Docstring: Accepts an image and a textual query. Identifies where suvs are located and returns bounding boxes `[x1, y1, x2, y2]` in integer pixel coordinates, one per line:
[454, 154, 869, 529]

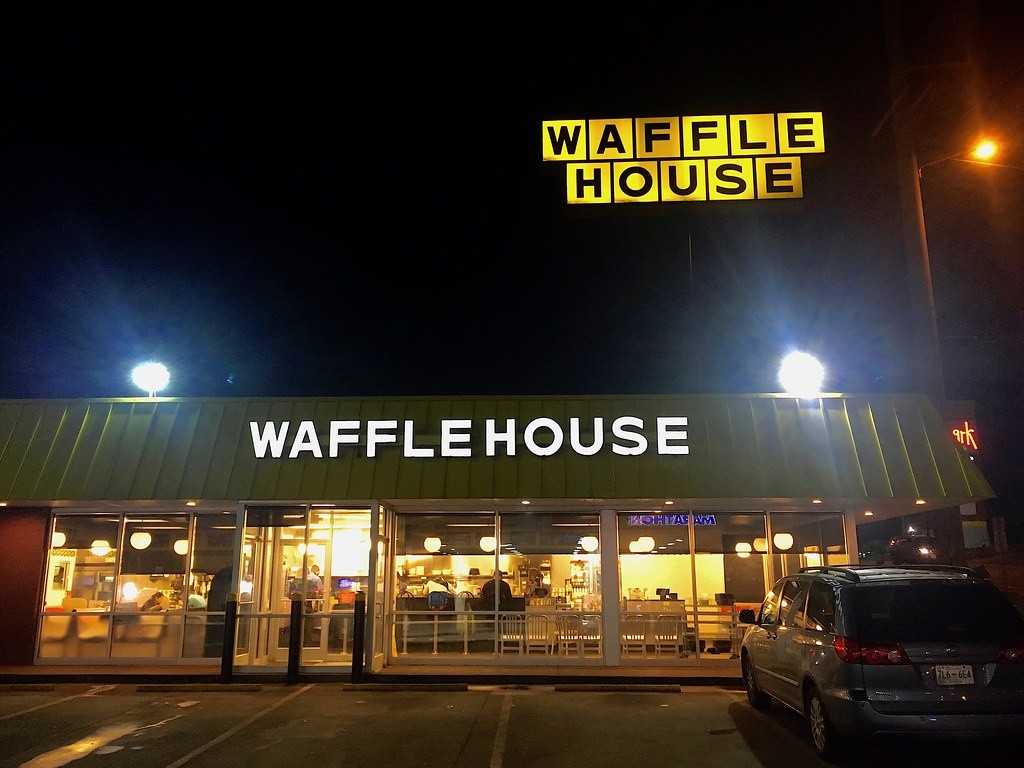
[740, 564, 1024, 760]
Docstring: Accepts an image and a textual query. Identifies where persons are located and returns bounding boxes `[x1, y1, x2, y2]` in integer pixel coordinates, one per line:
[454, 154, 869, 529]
[288, 567, 318, 601]
[178, 592, 205, 609]
[306, 565, 324, 596]
[422, 575, 457, 614]
[139, 592, 164, 613]
[480, 570, 513, 633]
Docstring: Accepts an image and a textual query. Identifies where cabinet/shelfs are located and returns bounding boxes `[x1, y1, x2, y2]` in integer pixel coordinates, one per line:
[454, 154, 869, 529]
[681, 603, 740, 659]
[593, 569, 621, 601]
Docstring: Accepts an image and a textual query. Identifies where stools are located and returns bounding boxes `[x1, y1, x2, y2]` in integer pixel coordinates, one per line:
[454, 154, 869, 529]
[456, 591, 474, 655]
[426, 591, 449, 655]
[397, 591, 415, 655]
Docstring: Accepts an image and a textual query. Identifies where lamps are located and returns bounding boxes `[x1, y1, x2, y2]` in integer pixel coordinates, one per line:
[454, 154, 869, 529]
[52, 531, 66, 549]
[130, 519, 151, 550]
[480, 524, 497, 552]
[173, 539, 188, 556]
[582, 524, 598, 552]
[423, 538, 440, 552]
[637, 536, 655, 552]
[735, 532, 793, 558]
[628, 540, 637, 552]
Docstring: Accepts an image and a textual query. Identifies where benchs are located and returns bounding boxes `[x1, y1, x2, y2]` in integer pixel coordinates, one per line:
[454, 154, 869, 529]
[41, 608, 169, 658]
[115, 602, 137, 608]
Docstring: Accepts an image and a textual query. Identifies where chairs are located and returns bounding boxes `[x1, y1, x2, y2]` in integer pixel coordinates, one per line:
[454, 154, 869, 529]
[654, 615, 679, 657]
[526, 615, 548, 654]
[501, 614, 523, 655]
[622, 615, 647, 658]
[580, 615, 602, 657]
[557, 615, 580, 657]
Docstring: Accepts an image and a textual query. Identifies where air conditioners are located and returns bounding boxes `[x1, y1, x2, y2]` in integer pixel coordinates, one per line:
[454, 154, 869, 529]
[89, 540, 111, 556]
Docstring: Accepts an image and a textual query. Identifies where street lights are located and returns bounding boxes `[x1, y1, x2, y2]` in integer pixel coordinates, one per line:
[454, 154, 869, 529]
[899, 132, 1008, 400]
